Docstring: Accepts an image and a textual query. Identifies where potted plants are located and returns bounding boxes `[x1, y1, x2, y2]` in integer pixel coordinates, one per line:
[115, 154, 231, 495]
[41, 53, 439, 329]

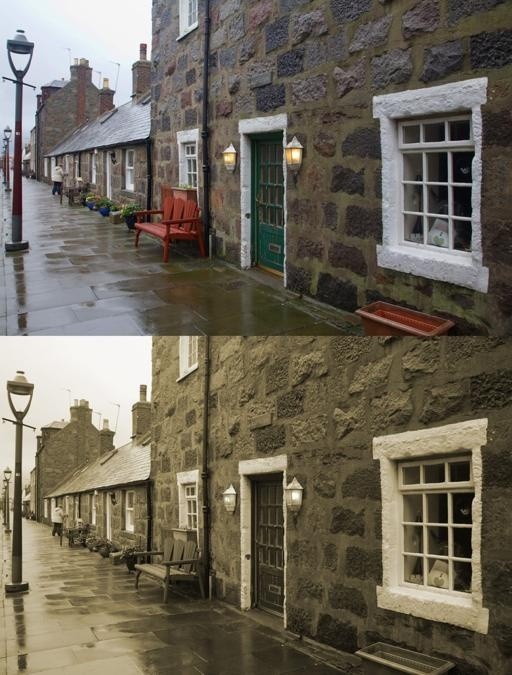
[79, 191, 141, 230]
[86, 536, 145, 575]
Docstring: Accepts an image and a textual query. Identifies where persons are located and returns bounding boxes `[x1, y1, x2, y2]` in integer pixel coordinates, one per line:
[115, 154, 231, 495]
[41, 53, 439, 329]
[51, 504, 63, 536]
[52, 162, 66, 196]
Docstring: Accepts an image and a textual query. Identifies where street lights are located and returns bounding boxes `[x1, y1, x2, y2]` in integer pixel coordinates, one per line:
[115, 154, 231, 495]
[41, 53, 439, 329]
[5, 592, 33, 675]
[2, 465, 12, 533]
[2, 124, 13, 193]
[1, 370, 39, 594]
[5, 250, 33, 336]
[0, 27, 37, 253]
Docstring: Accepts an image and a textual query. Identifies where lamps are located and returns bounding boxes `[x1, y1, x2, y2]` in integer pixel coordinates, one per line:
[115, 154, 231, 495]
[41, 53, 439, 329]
[284, 476, 304, 520]
[223, 483, 236, 515]
[285, 134, 303, 182]
[223, 141, 237, 173]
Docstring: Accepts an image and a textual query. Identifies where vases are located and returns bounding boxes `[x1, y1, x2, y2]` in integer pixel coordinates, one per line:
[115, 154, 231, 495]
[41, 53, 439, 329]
[357, 300, 456, 336]
[353, 641, 456, 675]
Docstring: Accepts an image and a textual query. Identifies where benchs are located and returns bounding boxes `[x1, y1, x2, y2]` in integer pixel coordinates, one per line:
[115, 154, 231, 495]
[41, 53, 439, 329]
[132, 197, 206, 263]
[134, 539, 206, 604]
